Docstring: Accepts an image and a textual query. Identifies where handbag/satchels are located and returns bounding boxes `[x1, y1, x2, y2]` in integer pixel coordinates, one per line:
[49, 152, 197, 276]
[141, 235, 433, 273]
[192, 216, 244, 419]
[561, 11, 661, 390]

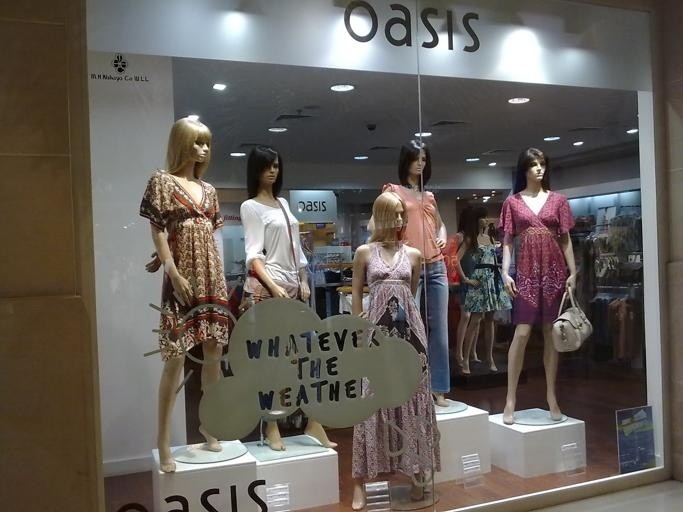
[551, 286, 593, 353]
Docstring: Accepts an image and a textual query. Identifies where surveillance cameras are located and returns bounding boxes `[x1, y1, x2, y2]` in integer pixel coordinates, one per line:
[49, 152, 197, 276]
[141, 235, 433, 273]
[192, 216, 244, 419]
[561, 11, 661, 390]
[367, 121, 377, 130]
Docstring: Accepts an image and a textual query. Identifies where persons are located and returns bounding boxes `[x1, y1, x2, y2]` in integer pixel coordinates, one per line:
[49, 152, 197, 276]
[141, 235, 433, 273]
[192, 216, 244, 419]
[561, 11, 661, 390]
[382, 141, 452, 408]
[453, 224, 482, 369]
[346, 190, 440, 511]
[455, 201, 501, 374]
[140, 115, 229, 474]
[496, 149, 578, 422]
[240, 142, 339, 449]
